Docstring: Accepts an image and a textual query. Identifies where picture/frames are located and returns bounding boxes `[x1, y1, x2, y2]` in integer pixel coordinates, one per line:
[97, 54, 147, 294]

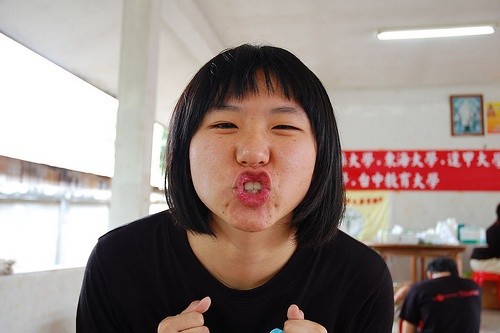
[450, 94, 484, 136]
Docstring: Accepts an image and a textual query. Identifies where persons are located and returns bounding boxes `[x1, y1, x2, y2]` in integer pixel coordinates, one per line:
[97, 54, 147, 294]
[397, 256, 481, 333]
[75, 43, 393, 333]
[486, 204, 500, 248]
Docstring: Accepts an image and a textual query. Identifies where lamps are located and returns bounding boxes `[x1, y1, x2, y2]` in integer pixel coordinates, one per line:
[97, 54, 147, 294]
[375, 21, 496, 41]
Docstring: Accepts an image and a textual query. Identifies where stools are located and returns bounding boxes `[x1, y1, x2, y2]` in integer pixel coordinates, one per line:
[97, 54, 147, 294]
[473, 271, 500, 311]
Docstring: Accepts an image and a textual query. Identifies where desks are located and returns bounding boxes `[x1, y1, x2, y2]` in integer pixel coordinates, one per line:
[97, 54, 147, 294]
[366, 243, 466, 286]
